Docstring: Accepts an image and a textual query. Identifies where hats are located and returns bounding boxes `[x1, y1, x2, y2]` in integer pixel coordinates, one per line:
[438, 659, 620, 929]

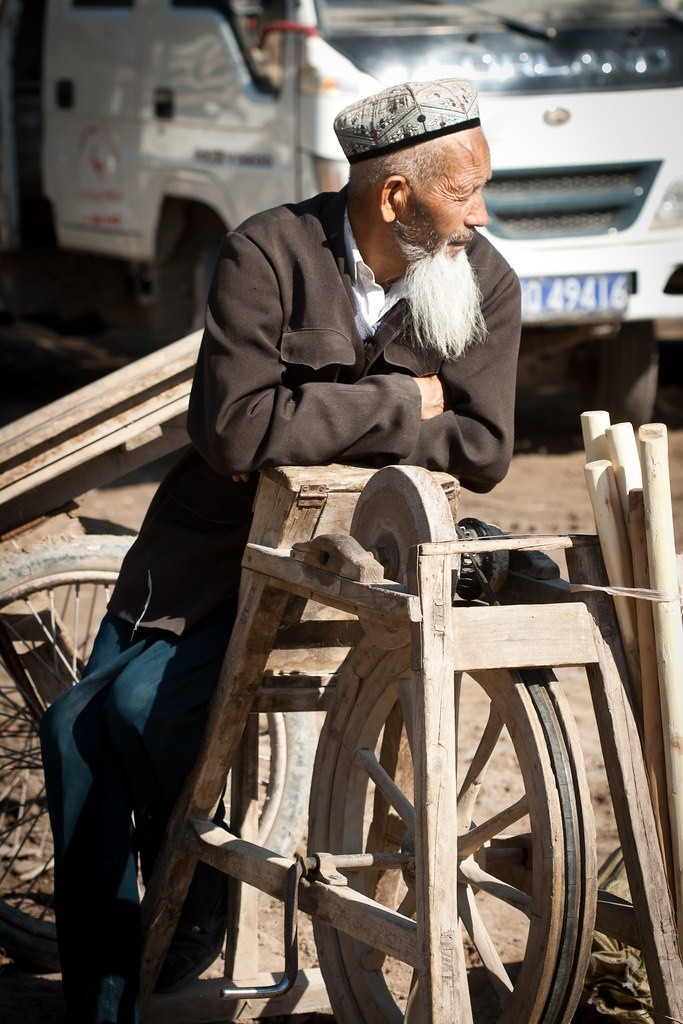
[333, 79, 480, 164]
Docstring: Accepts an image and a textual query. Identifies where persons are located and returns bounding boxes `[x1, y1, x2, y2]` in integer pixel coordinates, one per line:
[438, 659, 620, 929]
[38, 77, 522, 1024]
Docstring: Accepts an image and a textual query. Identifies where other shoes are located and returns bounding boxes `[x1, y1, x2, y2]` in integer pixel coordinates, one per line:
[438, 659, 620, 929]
[152, 830, 241, 994]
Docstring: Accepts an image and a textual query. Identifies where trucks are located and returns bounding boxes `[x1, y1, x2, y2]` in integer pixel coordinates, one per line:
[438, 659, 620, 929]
[2, 0, 683, 433]
